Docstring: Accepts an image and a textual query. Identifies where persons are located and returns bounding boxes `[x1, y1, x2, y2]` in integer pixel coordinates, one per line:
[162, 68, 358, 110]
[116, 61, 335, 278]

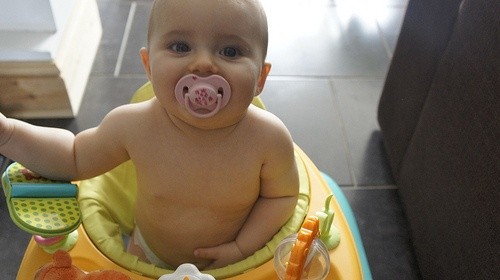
[0, 0, 299, 271]
